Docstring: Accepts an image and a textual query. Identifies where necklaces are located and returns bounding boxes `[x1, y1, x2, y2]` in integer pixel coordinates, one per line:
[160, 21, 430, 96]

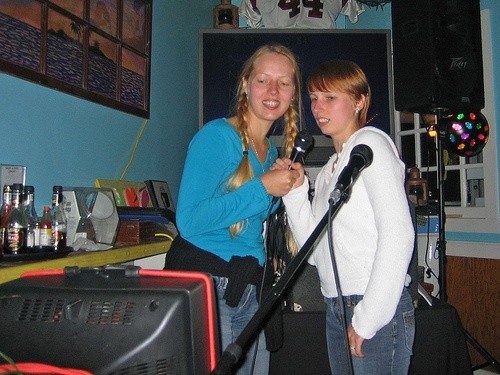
[249, 134, 269, 175]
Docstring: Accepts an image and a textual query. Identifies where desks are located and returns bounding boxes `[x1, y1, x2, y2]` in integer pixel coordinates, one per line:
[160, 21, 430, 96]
[269, 296, 474, 375]
[0, 241, 172, 284]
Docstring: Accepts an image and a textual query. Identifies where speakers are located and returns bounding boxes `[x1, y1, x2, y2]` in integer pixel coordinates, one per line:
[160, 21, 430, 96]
[391, 0, 485, 117]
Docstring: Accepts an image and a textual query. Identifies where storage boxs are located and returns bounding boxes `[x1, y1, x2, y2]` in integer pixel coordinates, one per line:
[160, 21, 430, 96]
[87, 220, 154, 244]
[144, 180, 176, 213]
[95, 178, 153, 207]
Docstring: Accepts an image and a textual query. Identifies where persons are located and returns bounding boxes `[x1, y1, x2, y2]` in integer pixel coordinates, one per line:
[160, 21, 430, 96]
[162, 44, 303, 374]
[270, 59, 415, 375]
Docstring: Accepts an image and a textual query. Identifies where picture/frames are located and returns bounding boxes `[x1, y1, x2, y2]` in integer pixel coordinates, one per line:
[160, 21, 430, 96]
[0, 0, 153, 120]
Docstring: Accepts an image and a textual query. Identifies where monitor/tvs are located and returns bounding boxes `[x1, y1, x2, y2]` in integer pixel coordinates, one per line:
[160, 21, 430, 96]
[0, 265, 221, 375]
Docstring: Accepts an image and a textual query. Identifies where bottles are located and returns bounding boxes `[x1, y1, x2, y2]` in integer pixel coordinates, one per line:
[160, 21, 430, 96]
[0, 185, 13, 254]
[40, 207, 52, 250]
[4, 184, 28, 255]
[51, 186, 67, 252]
[24, 186, 40, 252]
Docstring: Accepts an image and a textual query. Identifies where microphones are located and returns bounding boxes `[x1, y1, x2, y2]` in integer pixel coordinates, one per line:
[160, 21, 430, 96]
[328, 145, 373, 205]
[272, 131, 313, 204]
